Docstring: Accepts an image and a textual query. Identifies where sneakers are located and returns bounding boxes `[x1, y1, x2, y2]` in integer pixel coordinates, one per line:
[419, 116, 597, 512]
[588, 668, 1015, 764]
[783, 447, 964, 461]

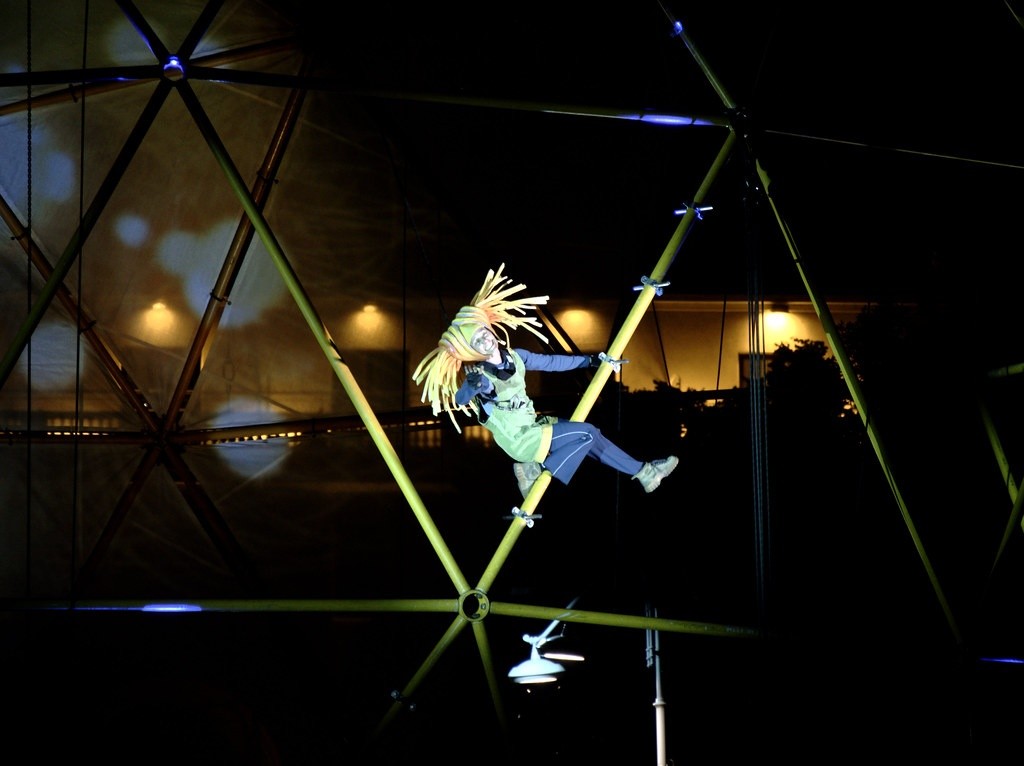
[514, 461, 542, 500]
[632, 456, 679, 493]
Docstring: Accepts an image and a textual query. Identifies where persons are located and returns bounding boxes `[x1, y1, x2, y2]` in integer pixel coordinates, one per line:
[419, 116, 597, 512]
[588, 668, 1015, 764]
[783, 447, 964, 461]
[415, 262, 680, 499]
